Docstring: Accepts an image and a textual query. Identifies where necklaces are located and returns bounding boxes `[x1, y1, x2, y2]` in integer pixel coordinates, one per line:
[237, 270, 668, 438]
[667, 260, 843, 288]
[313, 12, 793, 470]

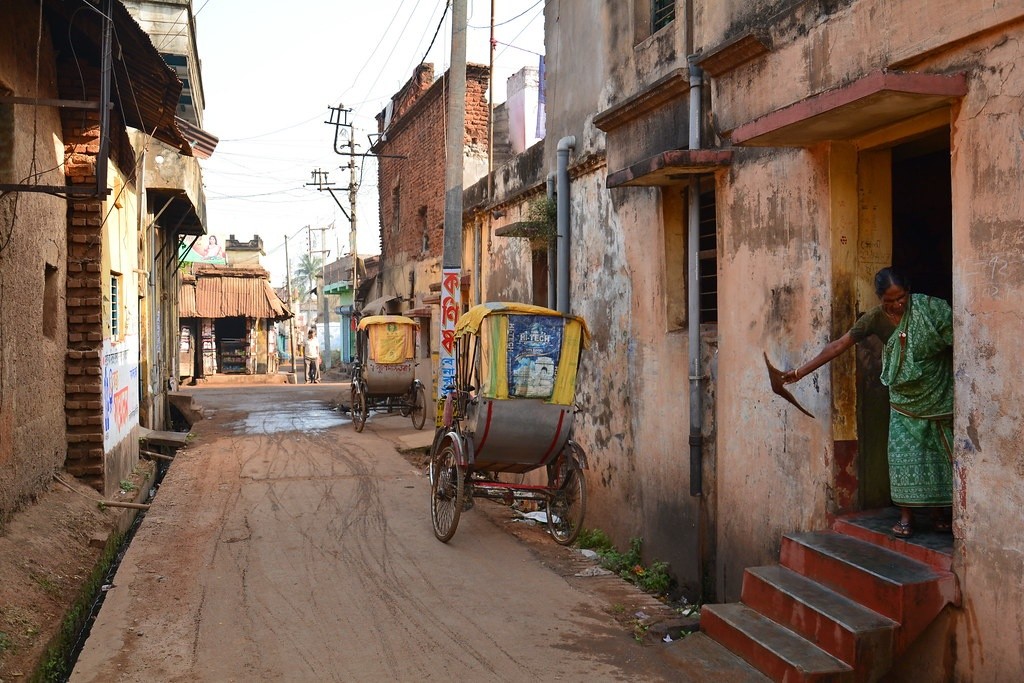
[890, 311, 911, 365]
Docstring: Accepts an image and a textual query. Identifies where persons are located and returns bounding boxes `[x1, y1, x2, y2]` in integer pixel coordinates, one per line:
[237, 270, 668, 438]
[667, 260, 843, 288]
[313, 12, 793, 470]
[302, 328, 321, 384]
[201, 235, 226, 265]
[780, 266, 953, 537]
[536, 366, 553, 385]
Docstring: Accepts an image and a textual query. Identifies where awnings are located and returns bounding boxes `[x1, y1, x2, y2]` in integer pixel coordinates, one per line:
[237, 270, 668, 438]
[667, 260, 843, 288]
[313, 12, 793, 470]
[147, 188, 206, 278]
[360, 295, 399, 316]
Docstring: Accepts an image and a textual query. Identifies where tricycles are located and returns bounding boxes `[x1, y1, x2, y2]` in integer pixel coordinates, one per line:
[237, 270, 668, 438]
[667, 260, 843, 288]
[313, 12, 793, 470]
[427, 302, 591, 545]
[349, 315, 429, 434]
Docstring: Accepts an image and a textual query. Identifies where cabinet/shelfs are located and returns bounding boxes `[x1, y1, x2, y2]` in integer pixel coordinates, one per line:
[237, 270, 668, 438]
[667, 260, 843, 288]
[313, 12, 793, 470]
[221, 338, 247, 373]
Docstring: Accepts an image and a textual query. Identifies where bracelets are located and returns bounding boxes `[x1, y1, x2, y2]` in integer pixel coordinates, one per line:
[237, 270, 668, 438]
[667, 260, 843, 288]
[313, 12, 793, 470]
[794, 368, 799, 378]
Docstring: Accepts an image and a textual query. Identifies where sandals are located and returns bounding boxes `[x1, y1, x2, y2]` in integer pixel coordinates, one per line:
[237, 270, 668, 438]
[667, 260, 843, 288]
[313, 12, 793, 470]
[931, 517, 951, 531]
[893, 517, 915, 538]
